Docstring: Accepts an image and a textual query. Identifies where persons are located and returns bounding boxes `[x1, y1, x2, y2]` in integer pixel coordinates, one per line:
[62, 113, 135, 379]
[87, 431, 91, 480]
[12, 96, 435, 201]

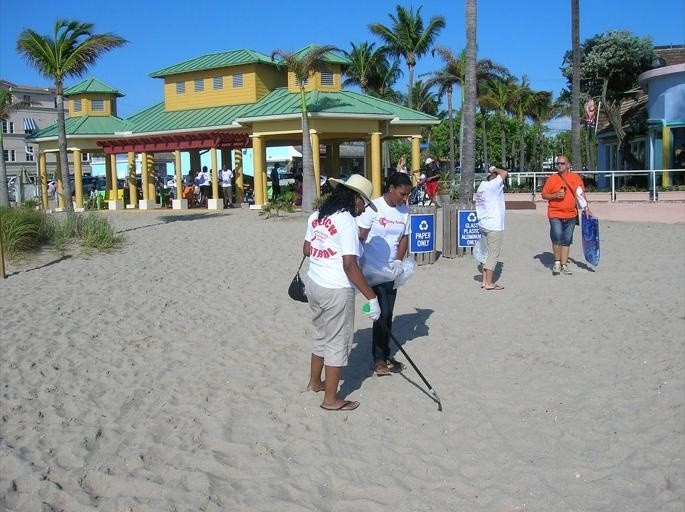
[397, 156, 409, 206]
[476, 166, 508, 291]
[88, 187, 100, 208]
[303, 174, 381, 411]
[542, 155, 593, 275]
[155, 163, 234, 209]
[270, 163, 280, 204]
[425, 157, 440, 208]
[355, 172, 413, 376]
[295, 178, 302, 206]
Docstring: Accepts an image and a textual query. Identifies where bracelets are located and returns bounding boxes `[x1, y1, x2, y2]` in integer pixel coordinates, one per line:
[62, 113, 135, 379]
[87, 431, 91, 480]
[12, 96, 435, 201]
[552, 194, 554, 199]
[584, 207, 589, 210]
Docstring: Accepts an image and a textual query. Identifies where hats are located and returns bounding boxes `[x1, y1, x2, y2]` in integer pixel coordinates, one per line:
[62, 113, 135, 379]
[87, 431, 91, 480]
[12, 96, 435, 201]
[328, 174, 377, 211]
[425, 157, 432, 165]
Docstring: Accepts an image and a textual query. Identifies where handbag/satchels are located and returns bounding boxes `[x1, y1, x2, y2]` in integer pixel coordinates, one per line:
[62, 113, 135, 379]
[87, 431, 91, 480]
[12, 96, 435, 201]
[582, 210, 599, 266]
[289, 270, 308, 302]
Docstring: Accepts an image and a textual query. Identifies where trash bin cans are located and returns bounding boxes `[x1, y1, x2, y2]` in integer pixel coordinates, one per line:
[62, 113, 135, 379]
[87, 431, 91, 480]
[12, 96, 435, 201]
[410, 204, 480, 265]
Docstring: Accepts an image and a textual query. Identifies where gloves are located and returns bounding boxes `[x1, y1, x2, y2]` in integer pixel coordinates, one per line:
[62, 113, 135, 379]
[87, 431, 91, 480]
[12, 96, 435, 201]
[365, 296, 382, 320]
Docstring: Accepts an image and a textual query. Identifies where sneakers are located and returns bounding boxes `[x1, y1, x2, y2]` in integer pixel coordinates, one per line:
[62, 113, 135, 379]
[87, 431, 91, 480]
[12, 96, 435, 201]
[553, 263, 572, 274]
[374, 358, 405, 375]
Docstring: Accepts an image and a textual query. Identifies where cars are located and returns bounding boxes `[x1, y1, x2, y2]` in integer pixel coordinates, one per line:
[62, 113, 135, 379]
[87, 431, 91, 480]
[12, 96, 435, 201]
[231, 175, 254, 198]
[70, 176, 175, 197]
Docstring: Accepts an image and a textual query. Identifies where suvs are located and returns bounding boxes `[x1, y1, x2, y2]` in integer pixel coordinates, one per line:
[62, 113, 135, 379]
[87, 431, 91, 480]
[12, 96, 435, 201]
[454, 166, 492, 181]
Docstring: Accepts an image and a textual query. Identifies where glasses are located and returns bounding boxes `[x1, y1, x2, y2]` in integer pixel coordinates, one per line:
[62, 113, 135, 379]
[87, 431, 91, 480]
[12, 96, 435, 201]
[556, 162, 565, 165]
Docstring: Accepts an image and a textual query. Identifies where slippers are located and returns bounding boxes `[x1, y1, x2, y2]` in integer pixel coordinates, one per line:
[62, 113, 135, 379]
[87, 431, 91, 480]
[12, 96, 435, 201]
[481, 284, 504, 290]
[320, 401, 359, 410]
